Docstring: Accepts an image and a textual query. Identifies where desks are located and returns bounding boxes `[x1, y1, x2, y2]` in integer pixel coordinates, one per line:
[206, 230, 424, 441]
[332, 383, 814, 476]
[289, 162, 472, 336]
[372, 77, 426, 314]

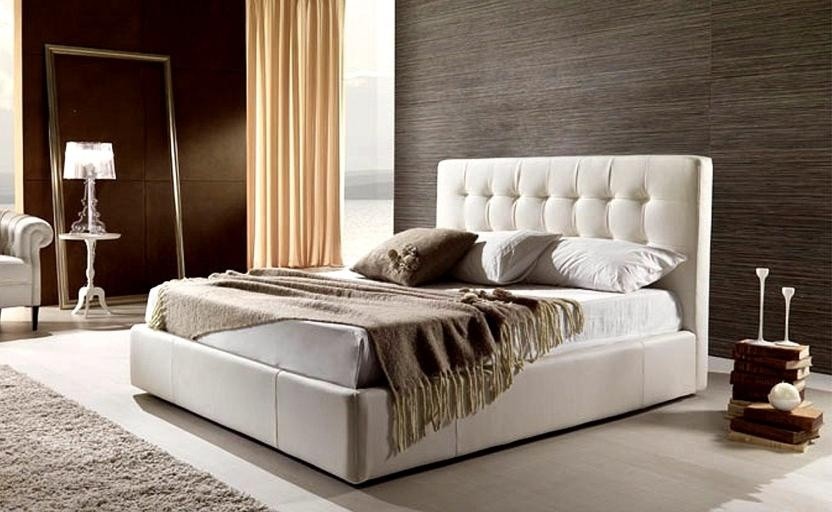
[58, 233, 122, 320]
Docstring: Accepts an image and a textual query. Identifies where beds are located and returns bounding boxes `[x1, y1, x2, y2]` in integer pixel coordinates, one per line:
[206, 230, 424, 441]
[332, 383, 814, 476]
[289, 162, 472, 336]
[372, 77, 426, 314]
[128, 154, 714, 487]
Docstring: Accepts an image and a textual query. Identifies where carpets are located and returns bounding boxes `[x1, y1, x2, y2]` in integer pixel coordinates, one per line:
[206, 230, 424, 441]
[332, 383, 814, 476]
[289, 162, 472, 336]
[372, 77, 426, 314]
[0, 363, 273, 512]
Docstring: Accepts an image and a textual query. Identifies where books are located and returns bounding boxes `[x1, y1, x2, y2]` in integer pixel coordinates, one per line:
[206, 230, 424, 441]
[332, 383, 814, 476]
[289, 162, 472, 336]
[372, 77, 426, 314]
[726, 337, 824, 453]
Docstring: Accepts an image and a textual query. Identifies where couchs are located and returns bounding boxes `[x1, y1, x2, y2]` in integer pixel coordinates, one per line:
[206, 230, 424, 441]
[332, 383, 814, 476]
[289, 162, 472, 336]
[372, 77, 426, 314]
[0, 208, 54, 334]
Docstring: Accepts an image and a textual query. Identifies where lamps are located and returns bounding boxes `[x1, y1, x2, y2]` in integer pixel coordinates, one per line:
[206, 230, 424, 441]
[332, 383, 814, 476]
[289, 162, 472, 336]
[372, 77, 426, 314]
[63, 142, 117, 233]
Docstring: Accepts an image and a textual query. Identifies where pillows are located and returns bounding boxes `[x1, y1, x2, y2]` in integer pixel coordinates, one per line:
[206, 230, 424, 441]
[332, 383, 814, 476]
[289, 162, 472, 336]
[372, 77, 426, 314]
[349, 228, 478, 287]
[525, 235, 689, 294]
[455, 230, 563, 285]
